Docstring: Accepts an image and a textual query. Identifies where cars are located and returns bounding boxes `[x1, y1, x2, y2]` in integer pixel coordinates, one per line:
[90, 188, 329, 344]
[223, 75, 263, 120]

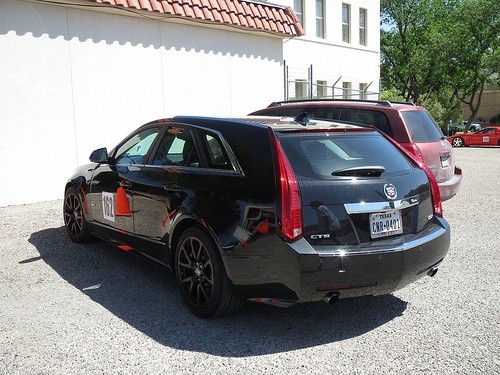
[445, 126, 499, 148]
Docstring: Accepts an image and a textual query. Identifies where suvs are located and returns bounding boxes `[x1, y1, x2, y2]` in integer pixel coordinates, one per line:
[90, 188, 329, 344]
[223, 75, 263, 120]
[62, 115, 453, 322]
[448, 120, 482, 133]
[246, 95, 462, 203]
